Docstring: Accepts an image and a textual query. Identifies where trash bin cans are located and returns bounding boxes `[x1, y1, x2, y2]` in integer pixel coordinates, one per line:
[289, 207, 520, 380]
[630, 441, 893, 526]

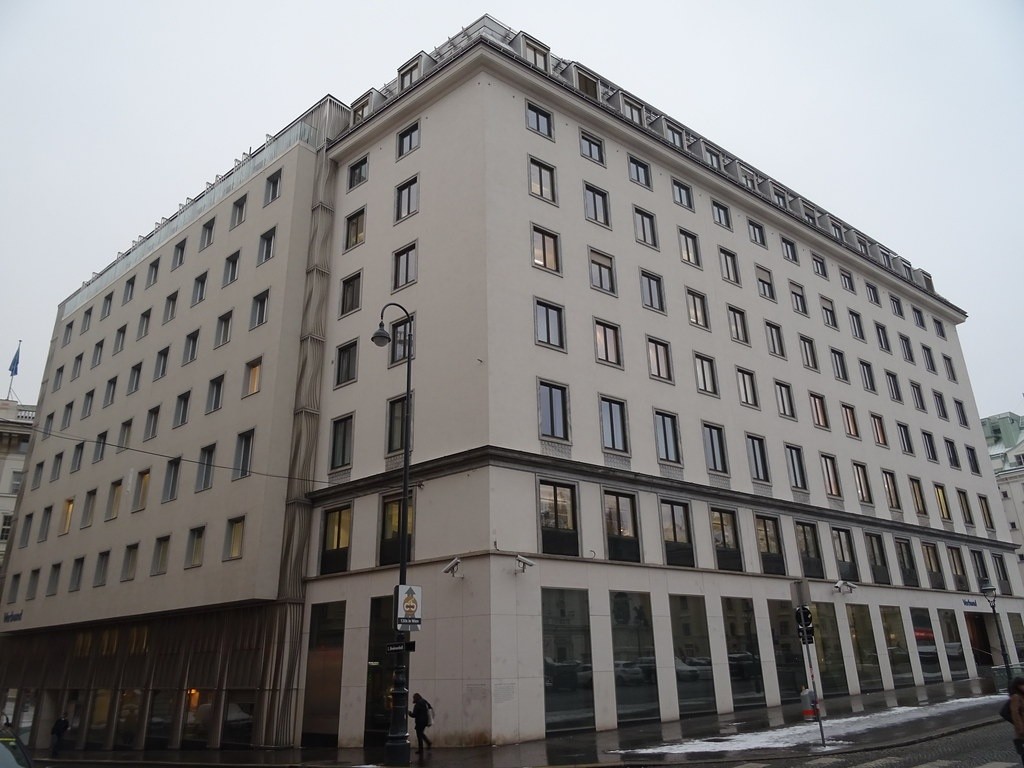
[800, 688, 820, 722]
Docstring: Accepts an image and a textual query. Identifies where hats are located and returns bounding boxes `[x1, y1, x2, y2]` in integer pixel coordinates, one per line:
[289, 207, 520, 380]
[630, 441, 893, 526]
[413, 693, 422, 702]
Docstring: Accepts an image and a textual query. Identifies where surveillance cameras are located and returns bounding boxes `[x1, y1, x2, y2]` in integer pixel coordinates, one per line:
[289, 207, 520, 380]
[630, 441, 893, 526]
[442, 558, 460, 573]
[515, 555, 535, 566]
[846, 582, 857, 588]
[835, 581, 843, 588]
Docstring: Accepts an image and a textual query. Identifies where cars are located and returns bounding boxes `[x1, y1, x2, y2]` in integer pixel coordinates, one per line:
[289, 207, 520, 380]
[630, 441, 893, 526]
[687, 652, 759, 681]
[579, 664, 594, 684]
[545, 655, 578, 690]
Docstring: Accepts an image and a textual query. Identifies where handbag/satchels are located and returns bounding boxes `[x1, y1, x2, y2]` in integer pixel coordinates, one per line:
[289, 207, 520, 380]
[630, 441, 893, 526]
[426, 701, 433, 728]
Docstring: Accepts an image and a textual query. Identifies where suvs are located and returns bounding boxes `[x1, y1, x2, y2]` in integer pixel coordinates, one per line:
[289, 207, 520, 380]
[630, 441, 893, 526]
[639, 656, 700, 681]
[614, 661, 643, 686]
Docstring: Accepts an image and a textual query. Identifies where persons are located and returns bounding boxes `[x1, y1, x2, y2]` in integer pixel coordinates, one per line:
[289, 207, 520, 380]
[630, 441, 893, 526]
[1008, 674, 1024, 768]
[50, 711, 70, 756]
[408, 693, 435, 754]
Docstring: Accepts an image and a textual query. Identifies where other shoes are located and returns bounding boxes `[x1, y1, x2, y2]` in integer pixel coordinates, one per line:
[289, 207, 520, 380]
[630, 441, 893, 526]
[415, 748, 424, 754]
[425, 741, 432, 750]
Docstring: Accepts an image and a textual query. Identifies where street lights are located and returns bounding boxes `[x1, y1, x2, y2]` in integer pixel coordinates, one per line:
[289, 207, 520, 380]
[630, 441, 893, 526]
[982, 577, 1013, 680]
[370, 304, 415, 768]
